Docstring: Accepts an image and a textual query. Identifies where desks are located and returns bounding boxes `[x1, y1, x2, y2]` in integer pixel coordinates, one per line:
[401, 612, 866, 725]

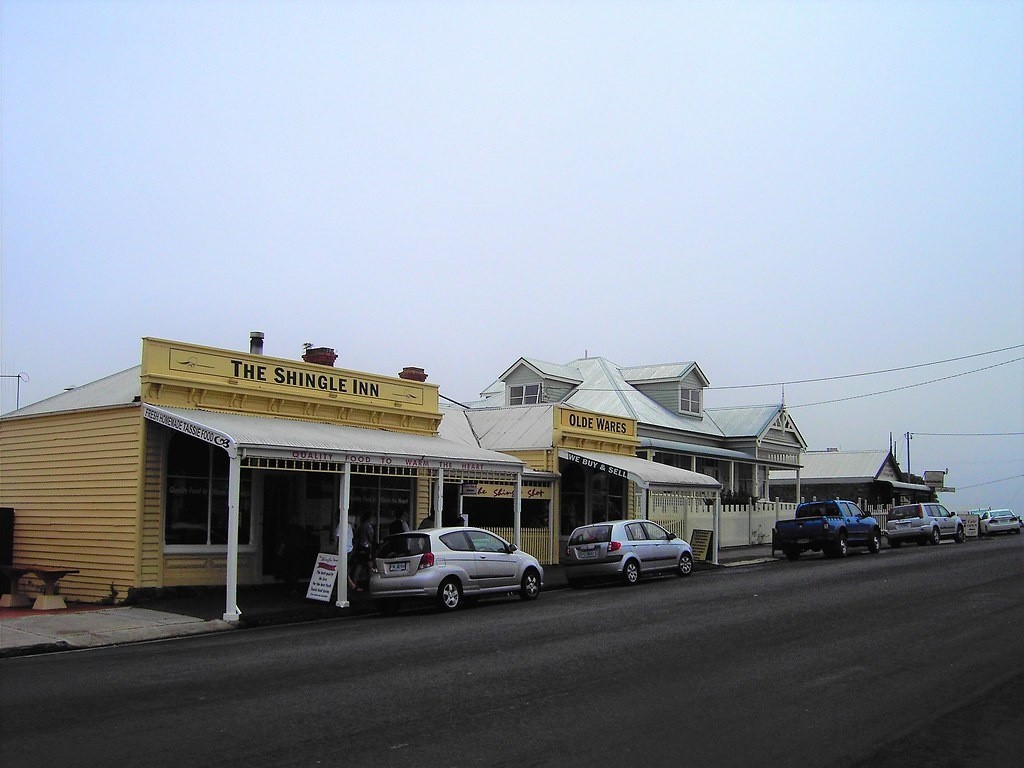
[0, 564, 80, 610]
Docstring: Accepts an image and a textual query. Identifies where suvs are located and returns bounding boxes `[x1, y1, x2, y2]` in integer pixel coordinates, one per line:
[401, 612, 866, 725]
[884, 503, 966, 546]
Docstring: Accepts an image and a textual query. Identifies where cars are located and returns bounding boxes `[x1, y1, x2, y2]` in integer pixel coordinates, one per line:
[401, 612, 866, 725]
[968, 509, 990, 521]
[564, 519, 695, 584]
[979, 509, 1020, 536]
[371, 527, 545, 610]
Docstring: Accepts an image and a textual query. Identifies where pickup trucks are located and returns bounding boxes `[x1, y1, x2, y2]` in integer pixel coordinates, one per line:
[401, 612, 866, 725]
[775, 500, 881, 558]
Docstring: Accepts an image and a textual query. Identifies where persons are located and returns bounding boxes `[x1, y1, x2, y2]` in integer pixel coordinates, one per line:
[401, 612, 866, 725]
[390, 510, 443, 554]
[335, 511, 376, 594]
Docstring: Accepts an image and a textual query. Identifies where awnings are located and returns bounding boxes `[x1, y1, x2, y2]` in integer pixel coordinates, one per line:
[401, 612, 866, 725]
[144, 401, 521, 621]
[558, 447, 725, 564]
[874, 479, 931, 494]
